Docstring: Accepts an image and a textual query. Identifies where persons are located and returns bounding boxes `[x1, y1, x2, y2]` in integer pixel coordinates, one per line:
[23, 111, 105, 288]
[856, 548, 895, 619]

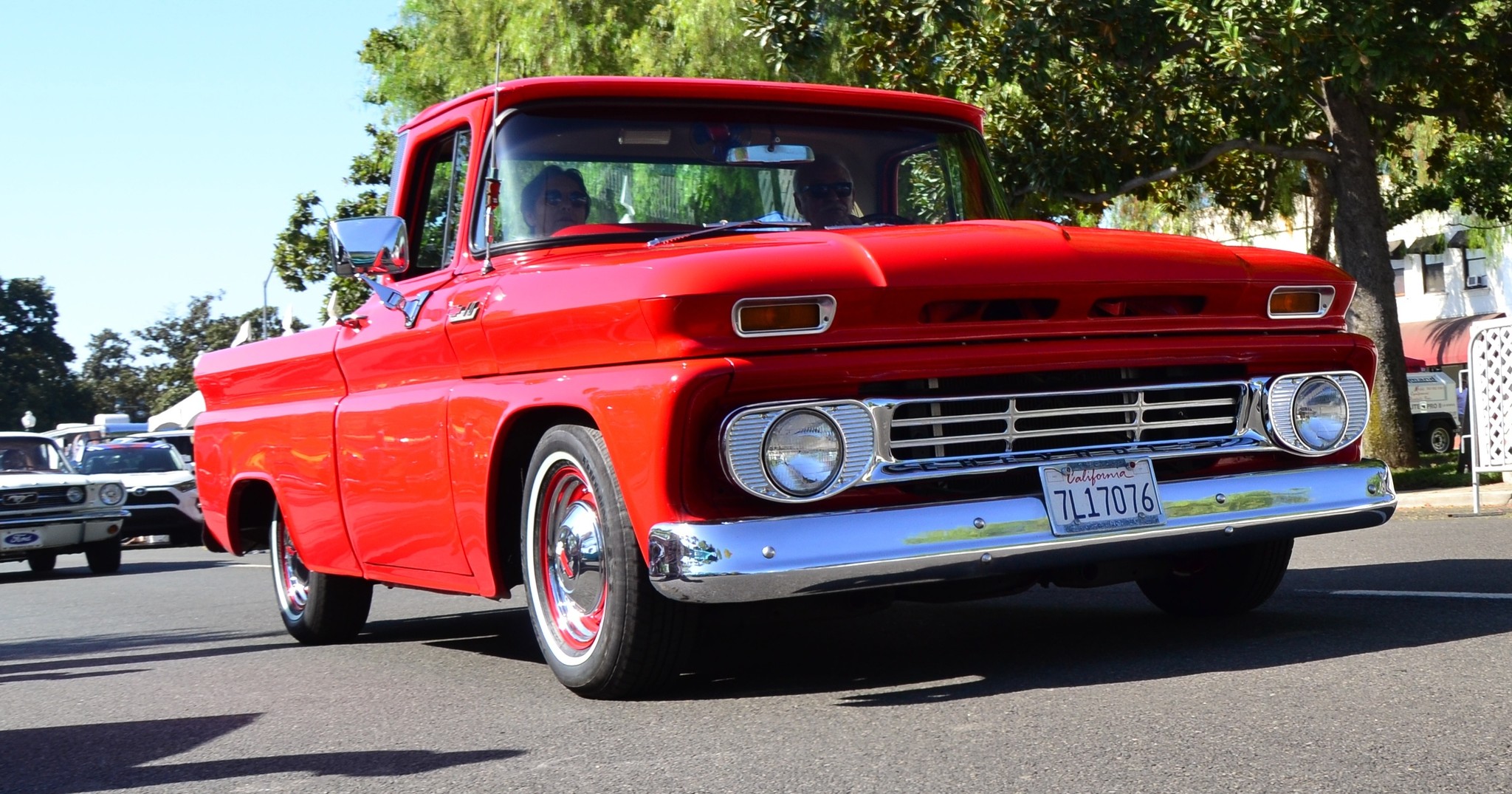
[791, 151, 865, 227]
[1456, 378, 1468, 430]
[520, 165, 592, 238]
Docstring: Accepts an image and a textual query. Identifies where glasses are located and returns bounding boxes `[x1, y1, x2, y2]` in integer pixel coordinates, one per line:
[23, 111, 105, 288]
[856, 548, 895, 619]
[798, 181, 852, 199]
[540, 190, 587, 209]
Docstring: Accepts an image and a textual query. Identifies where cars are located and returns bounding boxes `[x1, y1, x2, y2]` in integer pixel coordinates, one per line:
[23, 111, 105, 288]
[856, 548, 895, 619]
[0, 431, 132, 578]
[193, 77, 1399, 695]
[1406, 371, 1461, 454]
[109, 430, 198, 476]
[65, 435, 204, 544]
